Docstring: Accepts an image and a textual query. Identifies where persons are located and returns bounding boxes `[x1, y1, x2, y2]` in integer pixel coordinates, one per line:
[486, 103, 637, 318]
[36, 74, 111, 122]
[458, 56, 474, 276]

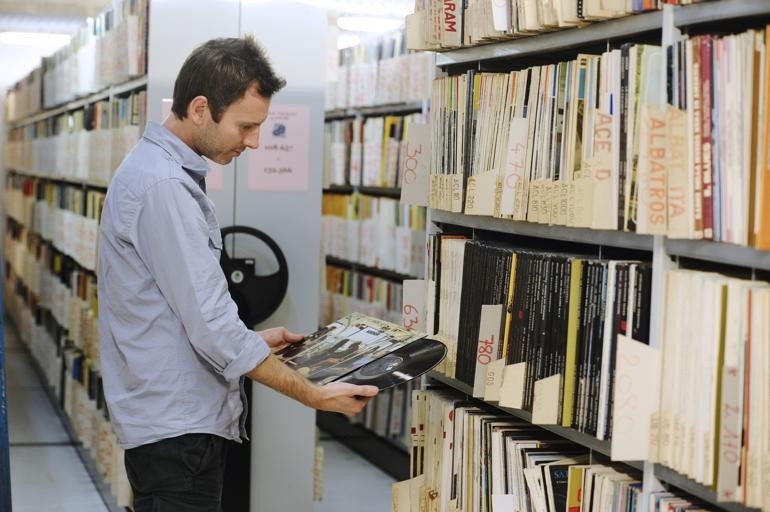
[299, 340, 362, 371]
[94, 32, 378, 512]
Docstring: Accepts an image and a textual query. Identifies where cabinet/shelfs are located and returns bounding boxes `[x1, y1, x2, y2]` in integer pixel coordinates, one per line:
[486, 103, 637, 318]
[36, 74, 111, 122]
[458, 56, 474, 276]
[421, 0, 770, 512]
[0, 1, 238, 508]
[315, 98, 424, 483]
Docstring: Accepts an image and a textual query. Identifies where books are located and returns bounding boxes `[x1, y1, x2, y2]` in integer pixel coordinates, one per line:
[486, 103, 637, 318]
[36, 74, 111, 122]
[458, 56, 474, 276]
[271, 310, 429, 390]
[1, 1, 152, 511]
[390, 0, 769, 510]
[316, 28, 443, 454]
